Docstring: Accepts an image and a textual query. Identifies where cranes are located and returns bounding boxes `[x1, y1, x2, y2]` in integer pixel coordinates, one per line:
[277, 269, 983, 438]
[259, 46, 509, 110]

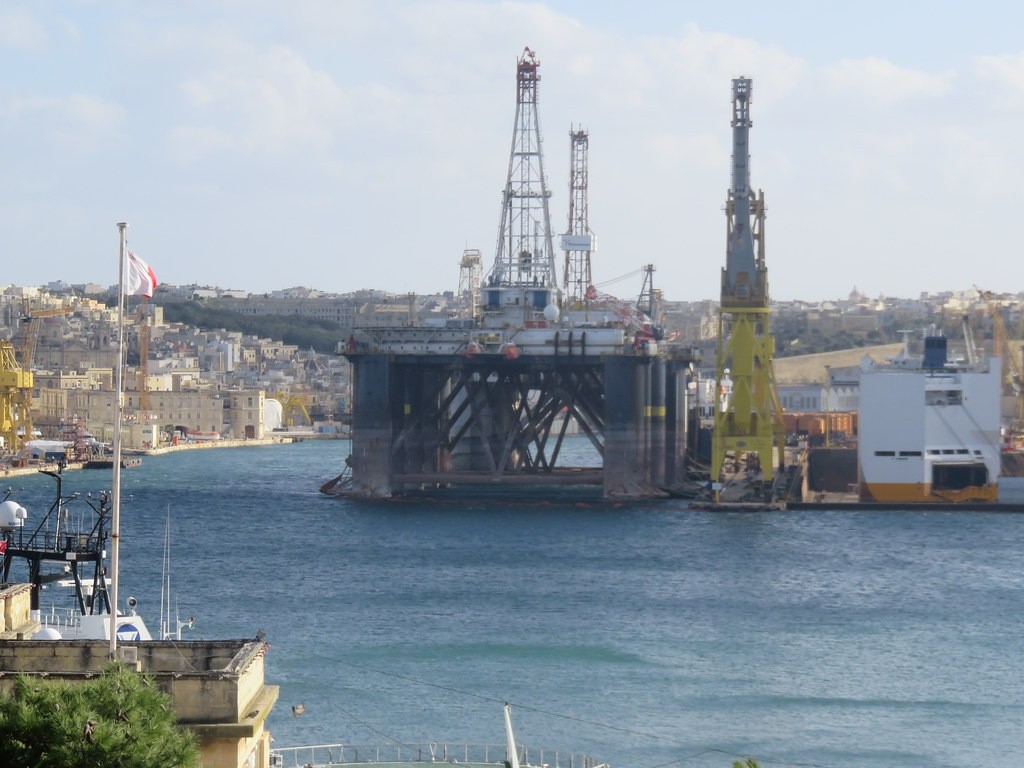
[583, 286, 682, 352]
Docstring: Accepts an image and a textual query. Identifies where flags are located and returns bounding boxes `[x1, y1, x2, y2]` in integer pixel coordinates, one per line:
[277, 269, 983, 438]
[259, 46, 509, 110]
[120, 249, 157, 298]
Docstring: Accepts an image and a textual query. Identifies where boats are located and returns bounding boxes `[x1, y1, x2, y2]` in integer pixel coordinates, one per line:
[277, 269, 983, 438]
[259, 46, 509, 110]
[186, 429, 220, 440]
[0, 450, 198, 643]
